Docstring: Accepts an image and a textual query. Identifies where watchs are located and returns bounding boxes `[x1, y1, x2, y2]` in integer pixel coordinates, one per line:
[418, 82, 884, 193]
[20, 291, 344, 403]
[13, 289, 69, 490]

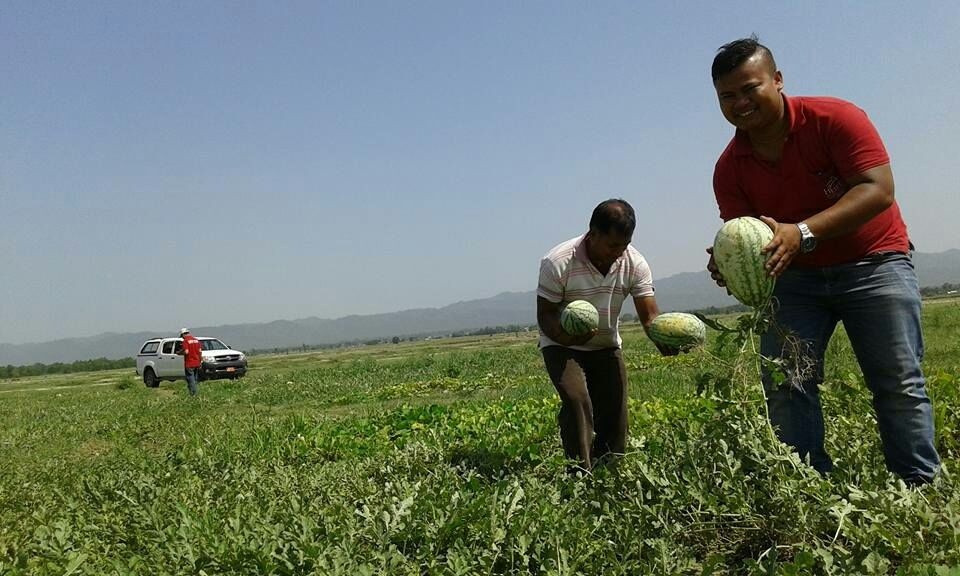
[795, 222, 818, 255]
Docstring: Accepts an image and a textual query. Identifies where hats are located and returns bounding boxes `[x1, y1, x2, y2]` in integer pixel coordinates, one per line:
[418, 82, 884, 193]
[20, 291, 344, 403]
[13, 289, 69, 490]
[179, 328, 190, 338]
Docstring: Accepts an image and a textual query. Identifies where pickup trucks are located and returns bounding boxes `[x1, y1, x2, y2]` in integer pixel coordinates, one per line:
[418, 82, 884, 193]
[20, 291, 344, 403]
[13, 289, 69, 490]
[136, 336, 247, 387]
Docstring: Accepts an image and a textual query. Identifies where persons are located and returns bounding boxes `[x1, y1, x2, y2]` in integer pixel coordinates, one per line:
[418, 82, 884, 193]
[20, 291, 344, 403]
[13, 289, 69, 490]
[176, 328, 203, 396]
[704, 38, 944, 489]
[534, 197, 691, 473]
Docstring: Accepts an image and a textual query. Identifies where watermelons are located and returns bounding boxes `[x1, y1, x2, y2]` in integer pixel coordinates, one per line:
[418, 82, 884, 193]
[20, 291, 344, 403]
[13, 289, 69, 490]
[713, 216, 777, 307]
[560, 300, 600, 334]
[649, 312, 705, 347]
